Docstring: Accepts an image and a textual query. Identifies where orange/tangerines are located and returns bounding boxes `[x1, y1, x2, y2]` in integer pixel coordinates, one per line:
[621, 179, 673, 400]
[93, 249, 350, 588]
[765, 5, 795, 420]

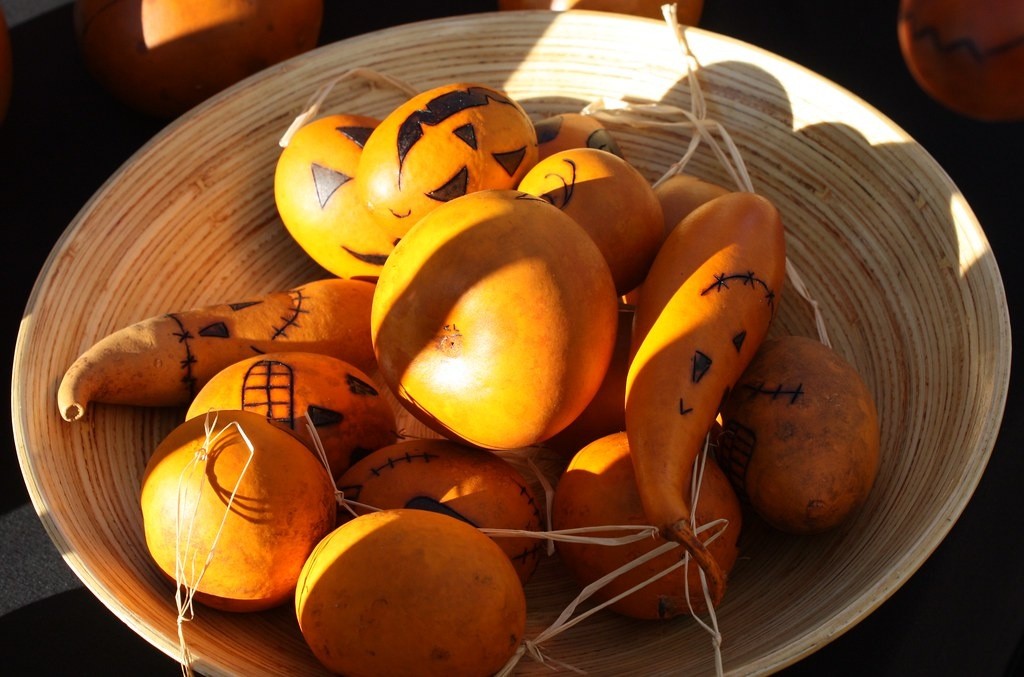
[141, 82, 881, 677]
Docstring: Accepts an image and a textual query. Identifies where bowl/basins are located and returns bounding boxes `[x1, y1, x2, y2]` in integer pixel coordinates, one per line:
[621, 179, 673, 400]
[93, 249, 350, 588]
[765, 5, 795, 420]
[9, 7, 1014, 677]
